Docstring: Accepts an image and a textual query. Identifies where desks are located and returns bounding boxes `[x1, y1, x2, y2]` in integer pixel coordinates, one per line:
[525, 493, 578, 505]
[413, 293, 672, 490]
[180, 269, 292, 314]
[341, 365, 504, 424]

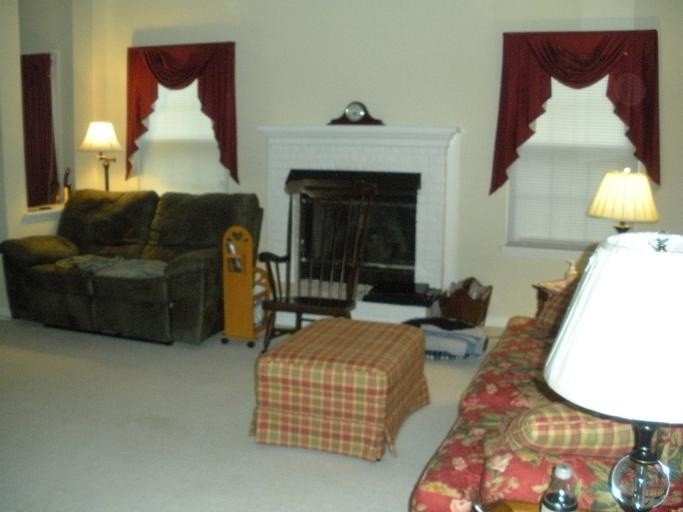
[252, 318, 436, 462]
[531, 276, 571, 322]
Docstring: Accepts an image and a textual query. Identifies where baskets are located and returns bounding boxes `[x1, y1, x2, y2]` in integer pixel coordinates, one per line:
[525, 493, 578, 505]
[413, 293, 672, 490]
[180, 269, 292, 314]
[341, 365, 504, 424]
[437, 276, 491, 324]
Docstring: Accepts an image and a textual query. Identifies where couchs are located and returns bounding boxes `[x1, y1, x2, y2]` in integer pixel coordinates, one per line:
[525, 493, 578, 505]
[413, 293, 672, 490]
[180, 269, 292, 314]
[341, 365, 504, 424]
[403, 262, 682, 512]
[1, 188, 265, 347]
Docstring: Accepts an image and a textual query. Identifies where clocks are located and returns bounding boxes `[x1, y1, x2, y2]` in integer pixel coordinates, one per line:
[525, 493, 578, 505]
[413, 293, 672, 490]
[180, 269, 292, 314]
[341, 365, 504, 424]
[327, 100, 385, 125]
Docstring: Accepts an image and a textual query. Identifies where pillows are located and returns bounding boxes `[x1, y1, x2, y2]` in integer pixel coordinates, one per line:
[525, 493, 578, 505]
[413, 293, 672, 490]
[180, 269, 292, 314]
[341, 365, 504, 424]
[498, 399, 638, 455]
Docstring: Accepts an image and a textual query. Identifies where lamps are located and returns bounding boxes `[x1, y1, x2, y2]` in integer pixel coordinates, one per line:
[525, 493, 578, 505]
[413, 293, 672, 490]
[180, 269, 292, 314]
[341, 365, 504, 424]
[80, 118, 120, 191]
[540, 227, 682, 512]
[581, 166, 662, 236]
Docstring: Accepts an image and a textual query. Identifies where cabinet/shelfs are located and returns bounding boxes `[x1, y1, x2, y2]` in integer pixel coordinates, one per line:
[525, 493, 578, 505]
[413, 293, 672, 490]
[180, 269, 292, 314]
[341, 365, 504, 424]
[220, 223, 275, 349]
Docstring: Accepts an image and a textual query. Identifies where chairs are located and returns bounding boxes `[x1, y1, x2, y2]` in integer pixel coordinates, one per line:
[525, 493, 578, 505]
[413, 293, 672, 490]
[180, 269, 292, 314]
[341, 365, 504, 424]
[254, 173, 376, 357]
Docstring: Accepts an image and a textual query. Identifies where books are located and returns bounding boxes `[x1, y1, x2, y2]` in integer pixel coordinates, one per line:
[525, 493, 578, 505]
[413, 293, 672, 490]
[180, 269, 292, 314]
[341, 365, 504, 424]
[349, 282, 442, 325]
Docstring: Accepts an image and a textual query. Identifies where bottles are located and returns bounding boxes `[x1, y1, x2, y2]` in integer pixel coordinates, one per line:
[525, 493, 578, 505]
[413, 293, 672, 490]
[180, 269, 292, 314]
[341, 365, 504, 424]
[538, 463, 578, 511]
